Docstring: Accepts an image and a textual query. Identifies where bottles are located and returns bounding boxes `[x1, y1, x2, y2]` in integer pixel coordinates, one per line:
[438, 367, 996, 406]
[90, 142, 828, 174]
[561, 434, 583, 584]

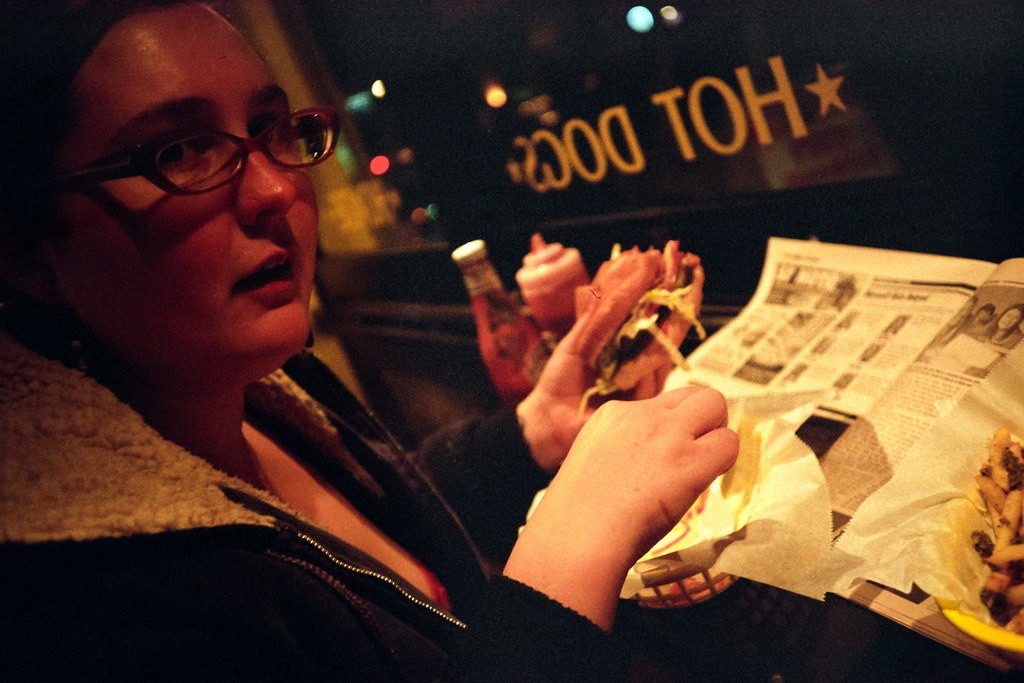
[516, 232, 590, 356]
[451, 239, 548, 406]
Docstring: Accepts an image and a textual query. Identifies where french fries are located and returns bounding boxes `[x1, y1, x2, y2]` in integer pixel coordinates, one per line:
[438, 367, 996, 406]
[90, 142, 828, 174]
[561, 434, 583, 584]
[973, 429, 1024, 635]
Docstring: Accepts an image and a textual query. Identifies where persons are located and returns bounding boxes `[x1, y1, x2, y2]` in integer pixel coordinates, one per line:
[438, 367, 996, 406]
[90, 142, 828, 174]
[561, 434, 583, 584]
[933, 295, 1024, 353]
[1, 0, 742, 683]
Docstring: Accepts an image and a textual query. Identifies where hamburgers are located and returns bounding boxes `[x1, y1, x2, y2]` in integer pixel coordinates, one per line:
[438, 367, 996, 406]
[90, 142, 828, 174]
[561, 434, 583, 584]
[573, 240, 706, 410]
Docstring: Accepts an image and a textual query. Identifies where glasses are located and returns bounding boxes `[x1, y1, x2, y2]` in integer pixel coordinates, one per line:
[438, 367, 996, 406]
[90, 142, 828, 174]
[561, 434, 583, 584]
[33, 104, 342, 194]
